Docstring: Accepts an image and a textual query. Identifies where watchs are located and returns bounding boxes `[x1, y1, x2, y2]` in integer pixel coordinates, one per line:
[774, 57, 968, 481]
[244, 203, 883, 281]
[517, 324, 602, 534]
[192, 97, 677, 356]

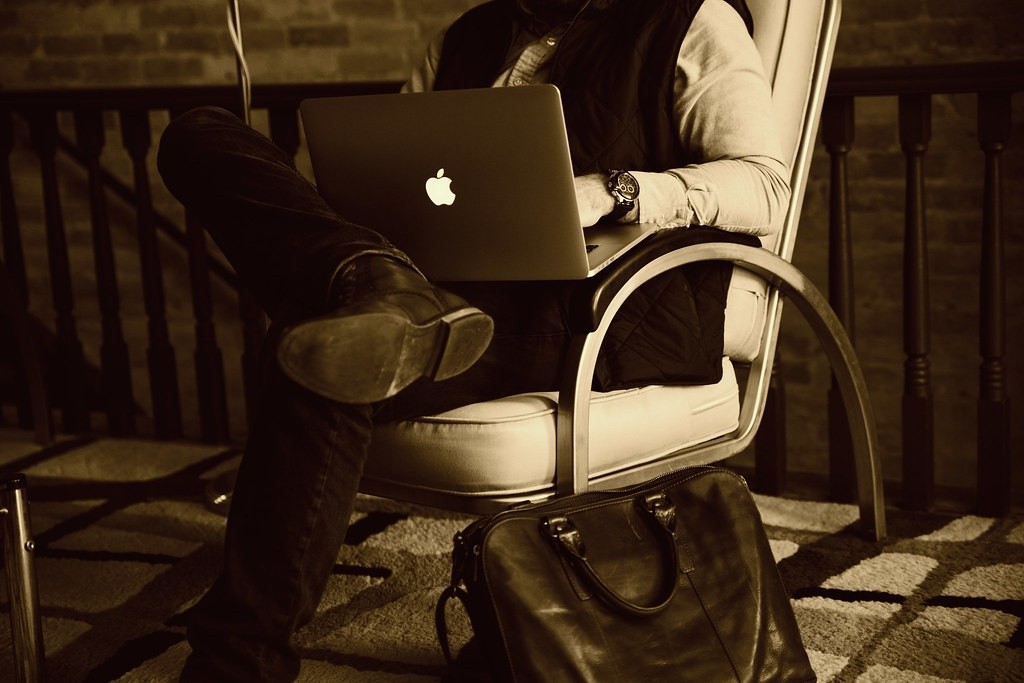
[607, 169, 640, 221]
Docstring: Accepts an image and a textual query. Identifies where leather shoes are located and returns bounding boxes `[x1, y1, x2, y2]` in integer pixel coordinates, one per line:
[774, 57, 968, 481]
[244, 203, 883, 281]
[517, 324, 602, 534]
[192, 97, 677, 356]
[273, 247, 494, 404]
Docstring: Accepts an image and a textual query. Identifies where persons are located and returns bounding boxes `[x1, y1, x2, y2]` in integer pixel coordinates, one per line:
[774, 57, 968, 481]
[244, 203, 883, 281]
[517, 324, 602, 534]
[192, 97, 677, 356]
[157, 0, 793, 683]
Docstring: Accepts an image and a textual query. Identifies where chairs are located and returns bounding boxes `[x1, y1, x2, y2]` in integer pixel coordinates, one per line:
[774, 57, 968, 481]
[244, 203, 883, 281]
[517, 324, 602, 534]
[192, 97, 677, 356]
[358, 0, 890, 542]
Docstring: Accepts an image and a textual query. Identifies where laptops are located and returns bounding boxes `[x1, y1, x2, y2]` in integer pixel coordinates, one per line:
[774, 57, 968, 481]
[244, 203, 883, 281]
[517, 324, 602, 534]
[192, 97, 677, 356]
[301, 84, 661, 281]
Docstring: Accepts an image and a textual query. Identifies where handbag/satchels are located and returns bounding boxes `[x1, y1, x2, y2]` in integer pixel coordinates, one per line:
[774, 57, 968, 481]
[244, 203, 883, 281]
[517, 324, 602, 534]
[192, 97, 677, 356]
[434, 466, 818, 683]
